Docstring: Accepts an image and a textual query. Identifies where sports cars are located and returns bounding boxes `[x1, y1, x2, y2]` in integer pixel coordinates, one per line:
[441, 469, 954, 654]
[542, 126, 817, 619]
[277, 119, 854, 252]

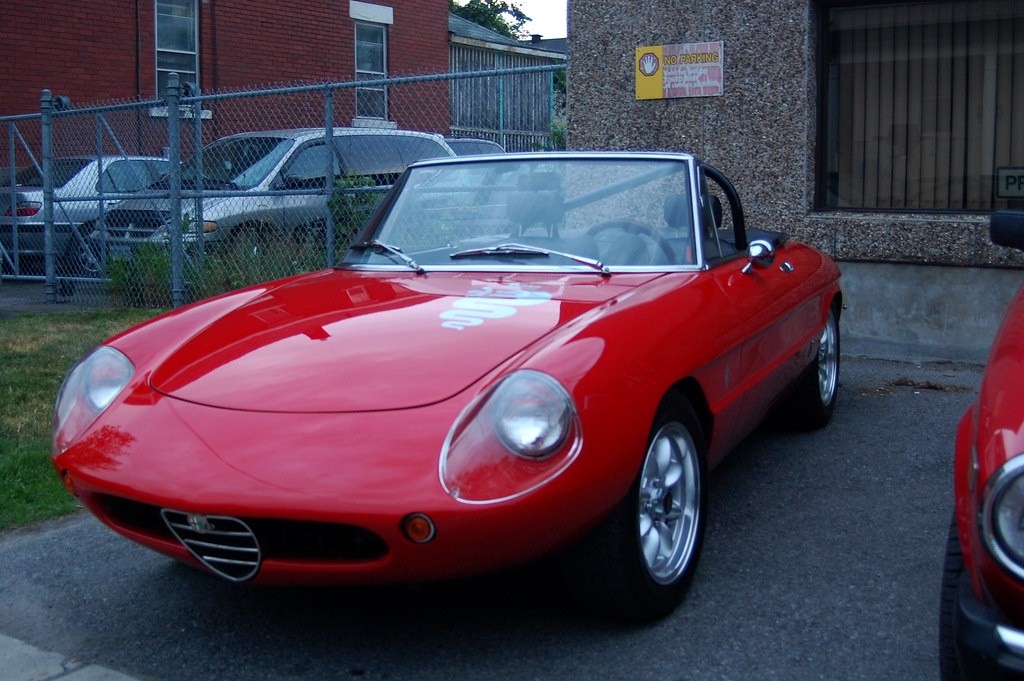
[938, 209, 1024, 681]
[50, 152, 845, 632]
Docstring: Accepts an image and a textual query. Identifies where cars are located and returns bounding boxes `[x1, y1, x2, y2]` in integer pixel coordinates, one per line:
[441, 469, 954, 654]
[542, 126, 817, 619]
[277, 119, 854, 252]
[445, 138, 532, 205]
[1, 154, 183, 286]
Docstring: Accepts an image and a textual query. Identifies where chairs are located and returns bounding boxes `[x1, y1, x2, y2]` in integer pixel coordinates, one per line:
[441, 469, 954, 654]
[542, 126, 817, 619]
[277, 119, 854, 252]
[654, 193, 735, 267]
[487, 190, 597, 265]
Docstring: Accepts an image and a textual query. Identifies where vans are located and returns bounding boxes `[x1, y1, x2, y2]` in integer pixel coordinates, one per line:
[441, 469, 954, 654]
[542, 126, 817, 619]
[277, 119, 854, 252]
[90, 127, 469, 275]
